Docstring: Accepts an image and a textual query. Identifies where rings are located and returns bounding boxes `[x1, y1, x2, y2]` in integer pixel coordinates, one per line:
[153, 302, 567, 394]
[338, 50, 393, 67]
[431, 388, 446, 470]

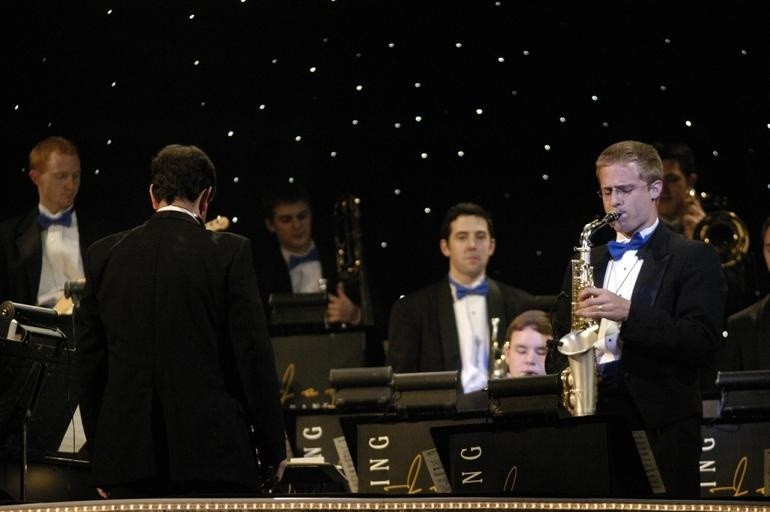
[597, 305, 603, 311]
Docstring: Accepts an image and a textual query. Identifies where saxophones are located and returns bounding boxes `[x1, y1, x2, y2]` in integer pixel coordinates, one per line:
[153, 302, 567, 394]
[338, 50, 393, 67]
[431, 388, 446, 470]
[561, 213, 620, 415]
[317, 195, 360, 330]
[490, 317, 532, 379]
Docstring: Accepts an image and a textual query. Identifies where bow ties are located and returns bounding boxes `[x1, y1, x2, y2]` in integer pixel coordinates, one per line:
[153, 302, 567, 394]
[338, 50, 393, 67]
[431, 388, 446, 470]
[288, 248, 318, 270]
[607, 232, 645, 261]
[456, 281, 489, 299]
[38, 211, 71, 231]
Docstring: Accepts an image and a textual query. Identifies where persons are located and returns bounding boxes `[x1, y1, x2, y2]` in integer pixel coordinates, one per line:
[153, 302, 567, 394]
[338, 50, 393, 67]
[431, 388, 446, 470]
[547, 140, 727, 498]
[73, 143, 289, 496]
[503, 303, 553, 384]
[246, 187, 384, 366]
[382, 200, 539, 399]
[721, 218, 770, 370]
[2, 135, 109, 457]
[649, 143, 709, 241]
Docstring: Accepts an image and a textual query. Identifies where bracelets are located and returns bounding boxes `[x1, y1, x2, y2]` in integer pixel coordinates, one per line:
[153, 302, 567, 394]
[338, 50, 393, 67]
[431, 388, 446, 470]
[351, 307, 363, 326]
